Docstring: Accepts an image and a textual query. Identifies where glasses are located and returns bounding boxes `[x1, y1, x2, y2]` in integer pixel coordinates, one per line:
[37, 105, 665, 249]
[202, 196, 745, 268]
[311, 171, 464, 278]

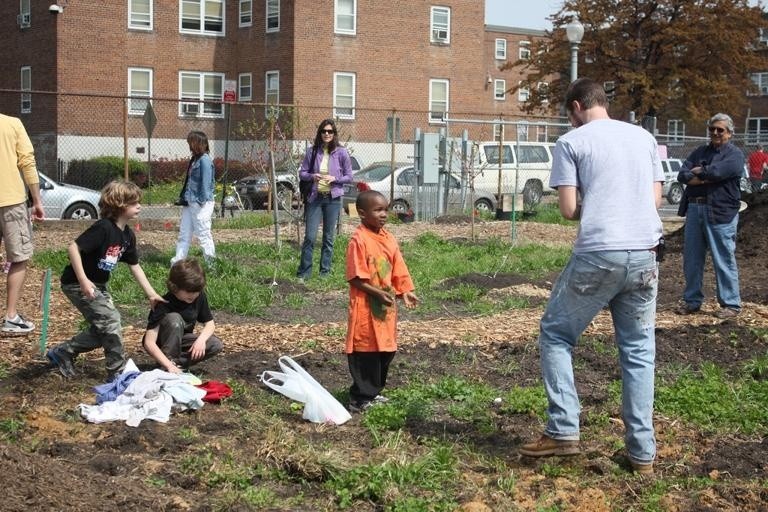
[709, 126, 729, 133]
[321, 130, 335, 135]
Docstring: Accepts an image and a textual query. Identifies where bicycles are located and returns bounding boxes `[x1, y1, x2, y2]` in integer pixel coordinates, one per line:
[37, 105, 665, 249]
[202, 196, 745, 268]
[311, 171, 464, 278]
[211, 180, 253, 219]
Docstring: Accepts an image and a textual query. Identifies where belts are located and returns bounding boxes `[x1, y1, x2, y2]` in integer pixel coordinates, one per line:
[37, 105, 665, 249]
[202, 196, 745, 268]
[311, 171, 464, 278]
[688, 197, 707, 203]
[316, 190, 331, 196]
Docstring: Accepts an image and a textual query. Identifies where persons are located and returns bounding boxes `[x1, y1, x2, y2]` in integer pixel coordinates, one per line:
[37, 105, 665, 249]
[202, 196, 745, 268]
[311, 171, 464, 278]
[344, 190, 420, 415]
[169, 131, 215, 276]
[0, 113, 46, 333]
[747, 143, 768, 204]
[674, 113, 745, 318]
[142, 258, 223, 375]
[518, 77, 666, 476]
[46, 179, 169, 382]
[295, 119, 353, 285]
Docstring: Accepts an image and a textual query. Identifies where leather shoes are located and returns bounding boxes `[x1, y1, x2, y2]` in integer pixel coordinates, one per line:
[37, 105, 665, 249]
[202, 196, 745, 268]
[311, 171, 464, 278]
[714, 309, 740, 318]
[676, 305, 698, 314]
[615, 447, 654, 474]
[519, 433, 580, 455]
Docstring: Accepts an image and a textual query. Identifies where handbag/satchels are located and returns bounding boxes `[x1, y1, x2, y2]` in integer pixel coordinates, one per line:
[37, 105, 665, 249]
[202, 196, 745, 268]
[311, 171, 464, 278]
[299, 145, 319, 204]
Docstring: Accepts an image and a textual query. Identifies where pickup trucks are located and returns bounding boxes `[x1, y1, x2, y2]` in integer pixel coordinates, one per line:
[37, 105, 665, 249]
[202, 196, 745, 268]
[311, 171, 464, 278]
[466, 141, 557, 207]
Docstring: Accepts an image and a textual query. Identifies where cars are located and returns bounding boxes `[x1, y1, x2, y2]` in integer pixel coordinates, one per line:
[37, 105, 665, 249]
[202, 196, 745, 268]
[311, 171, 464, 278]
[26, 169, 102, 220]
[343, 161, 498, 224]
[236, 153, 366, 210]
[660, 158, 687, 205]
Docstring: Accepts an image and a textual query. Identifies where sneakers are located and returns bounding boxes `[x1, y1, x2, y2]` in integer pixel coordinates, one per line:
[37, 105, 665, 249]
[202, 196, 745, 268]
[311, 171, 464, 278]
[372, 396, 388, 404]
[106, 373, 124, 383]
[296, 277, 308, 285]
[349, 400, 373, 414]
[2, 315, 34, 333]
[47, 349, 74, 378]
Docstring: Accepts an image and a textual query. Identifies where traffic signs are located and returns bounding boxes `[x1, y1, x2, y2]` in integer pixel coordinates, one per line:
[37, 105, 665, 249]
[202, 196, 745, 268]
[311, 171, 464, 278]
[565, 14, 584, 131]
